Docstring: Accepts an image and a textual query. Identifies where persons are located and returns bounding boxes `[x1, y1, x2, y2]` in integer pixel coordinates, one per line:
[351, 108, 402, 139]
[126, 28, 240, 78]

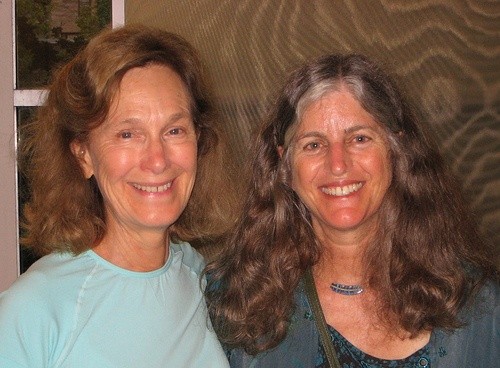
[199, 51, 500, 368]
[0, 22, 231, 368]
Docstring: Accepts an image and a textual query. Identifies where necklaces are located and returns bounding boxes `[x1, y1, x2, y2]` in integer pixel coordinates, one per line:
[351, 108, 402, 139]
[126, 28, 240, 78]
[323, 275, 364, 295]
